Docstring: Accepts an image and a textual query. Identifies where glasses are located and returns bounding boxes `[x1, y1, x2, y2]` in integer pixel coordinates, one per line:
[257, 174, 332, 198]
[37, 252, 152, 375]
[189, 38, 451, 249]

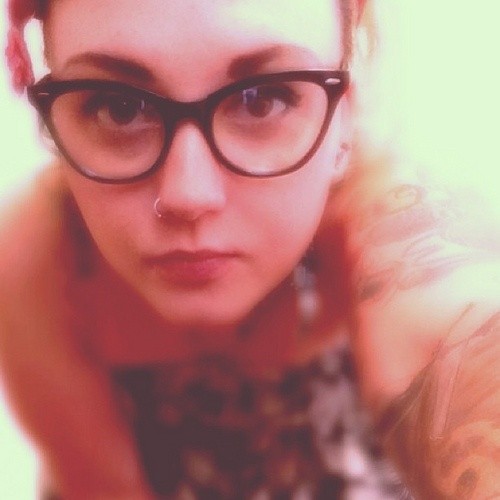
[28, 70, 350, 176]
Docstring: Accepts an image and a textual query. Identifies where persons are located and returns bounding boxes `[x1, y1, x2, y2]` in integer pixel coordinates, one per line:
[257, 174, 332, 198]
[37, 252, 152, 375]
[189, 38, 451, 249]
[2, 1, 498, 500]
